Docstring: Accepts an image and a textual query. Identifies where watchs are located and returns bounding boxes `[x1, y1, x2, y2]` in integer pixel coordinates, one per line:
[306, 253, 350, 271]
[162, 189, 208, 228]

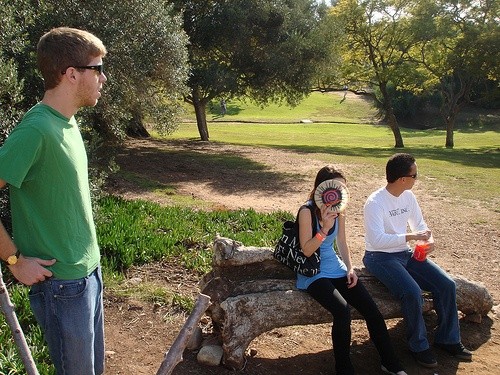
[4, 250, 20, 265]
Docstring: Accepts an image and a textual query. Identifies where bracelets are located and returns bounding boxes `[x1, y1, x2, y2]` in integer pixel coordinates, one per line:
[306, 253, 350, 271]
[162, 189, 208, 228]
[316, 233, 326, 241]
[321, 229, 328, 235]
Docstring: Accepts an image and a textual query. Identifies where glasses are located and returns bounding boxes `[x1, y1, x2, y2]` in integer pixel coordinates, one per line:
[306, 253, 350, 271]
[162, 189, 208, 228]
[61, 66, 105, 75]
[402, 174, 418, 179]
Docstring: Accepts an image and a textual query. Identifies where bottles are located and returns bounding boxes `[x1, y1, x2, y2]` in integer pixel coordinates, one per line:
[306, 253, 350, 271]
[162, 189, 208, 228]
[413, 230, 431, 261]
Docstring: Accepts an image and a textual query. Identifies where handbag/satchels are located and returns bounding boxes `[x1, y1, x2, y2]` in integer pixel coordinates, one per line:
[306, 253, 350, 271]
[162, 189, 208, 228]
[274, 206, 321, 277]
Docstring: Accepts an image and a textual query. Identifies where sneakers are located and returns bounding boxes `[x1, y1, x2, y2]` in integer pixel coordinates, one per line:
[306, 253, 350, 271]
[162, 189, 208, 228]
[434, 340, 471, 359]
[411, 350, 437, 368]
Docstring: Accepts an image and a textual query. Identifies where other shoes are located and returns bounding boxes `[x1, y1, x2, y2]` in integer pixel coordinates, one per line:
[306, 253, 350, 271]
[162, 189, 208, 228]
[382, 365, 408, 375]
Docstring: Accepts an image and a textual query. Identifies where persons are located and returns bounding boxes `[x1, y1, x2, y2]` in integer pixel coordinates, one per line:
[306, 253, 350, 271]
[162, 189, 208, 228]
[362, 152, 472, 367]
[297, 164, 409, 375]
[0, 27, 108, 375]
